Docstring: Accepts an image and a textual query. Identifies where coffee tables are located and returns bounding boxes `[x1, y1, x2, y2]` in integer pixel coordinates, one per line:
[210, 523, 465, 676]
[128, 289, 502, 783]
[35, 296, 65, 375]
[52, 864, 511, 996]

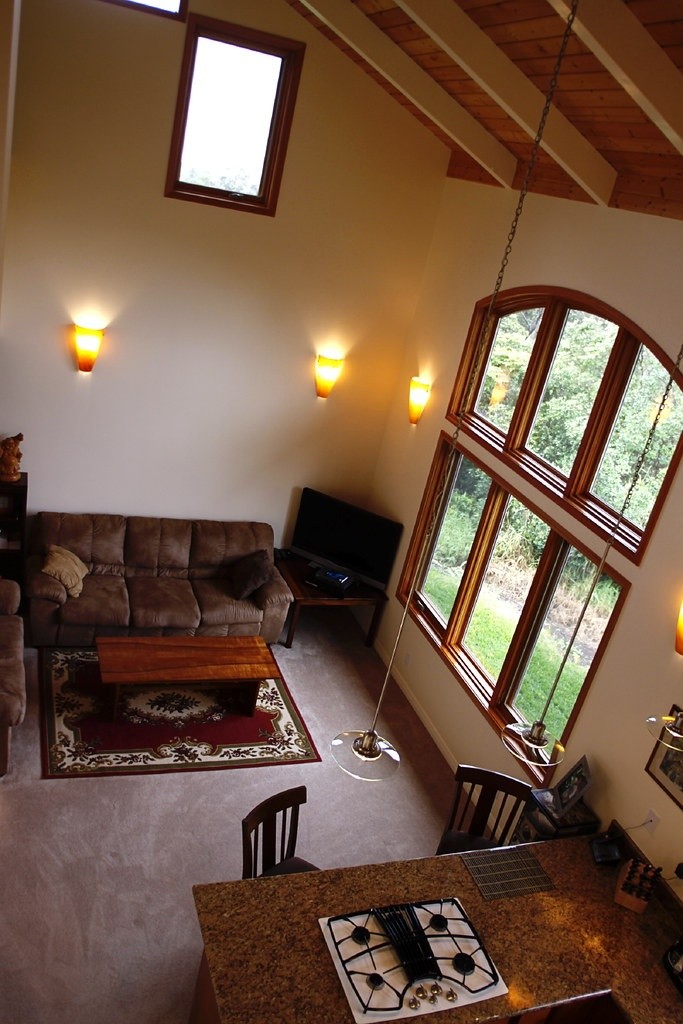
[94, 636, 282, 719]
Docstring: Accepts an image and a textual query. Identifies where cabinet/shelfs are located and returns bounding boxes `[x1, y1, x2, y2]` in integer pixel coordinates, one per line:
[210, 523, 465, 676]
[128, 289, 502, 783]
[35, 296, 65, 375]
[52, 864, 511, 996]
[1, 470, 28, 615]
[507, 788, 600, 845]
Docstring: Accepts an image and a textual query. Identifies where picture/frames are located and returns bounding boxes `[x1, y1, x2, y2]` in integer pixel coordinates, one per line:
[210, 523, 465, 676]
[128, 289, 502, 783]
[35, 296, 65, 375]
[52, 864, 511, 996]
[554, 754, 593, 817]
[644, 705, 683, 812]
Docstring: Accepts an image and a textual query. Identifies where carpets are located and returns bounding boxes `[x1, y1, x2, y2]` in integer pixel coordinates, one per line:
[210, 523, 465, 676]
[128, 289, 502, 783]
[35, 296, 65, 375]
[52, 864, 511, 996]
[37, 644, 322, 780]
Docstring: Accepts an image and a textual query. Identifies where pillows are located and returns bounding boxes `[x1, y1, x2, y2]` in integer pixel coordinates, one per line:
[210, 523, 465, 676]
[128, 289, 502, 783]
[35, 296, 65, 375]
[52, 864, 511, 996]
[41, 542, 88, 598]
[227, 549, 273, 601]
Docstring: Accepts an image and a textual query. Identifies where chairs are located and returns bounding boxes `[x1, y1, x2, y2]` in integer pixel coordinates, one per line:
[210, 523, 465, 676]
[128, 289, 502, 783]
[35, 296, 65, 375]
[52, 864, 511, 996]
[242, 786, 321, 880]
[435, 763, 533, 854]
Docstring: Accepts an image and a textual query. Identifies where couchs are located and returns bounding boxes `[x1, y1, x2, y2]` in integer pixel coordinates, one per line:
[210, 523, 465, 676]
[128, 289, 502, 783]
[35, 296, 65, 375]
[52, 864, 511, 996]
[0, 579, 27, 776]
[25, 510, 296, 649]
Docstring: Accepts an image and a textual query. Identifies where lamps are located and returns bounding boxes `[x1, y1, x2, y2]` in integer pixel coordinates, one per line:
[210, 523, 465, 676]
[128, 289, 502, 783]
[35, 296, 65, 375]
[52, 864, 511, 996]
[73, 325, 104, 373]
[501, 346, 683, 766]
[315, 354, 344, 400]
[329, 0, 578, 781]
[408, 377, 432, 426]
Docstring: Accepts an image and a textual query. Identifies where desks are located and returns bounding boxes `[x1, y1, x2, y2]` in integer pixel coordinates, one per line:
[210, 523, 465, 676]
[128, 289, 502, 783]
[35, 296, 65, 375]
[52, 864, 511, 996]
[273, 549, 389, 652]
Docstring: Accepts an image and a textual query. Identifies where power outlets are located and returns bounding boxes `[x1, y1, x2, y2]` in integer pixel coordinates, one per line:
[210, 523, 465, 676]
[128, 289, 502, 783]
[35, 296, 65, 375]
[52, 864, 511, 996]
[642, 809, 661, 832]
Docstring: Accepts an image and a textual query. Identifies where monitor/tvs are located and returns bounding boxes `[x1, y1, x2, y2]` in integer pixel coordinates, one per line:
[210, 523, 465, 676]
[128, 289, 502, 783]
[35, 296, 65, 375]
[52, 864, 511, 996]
[290, 487, 404, 592]
